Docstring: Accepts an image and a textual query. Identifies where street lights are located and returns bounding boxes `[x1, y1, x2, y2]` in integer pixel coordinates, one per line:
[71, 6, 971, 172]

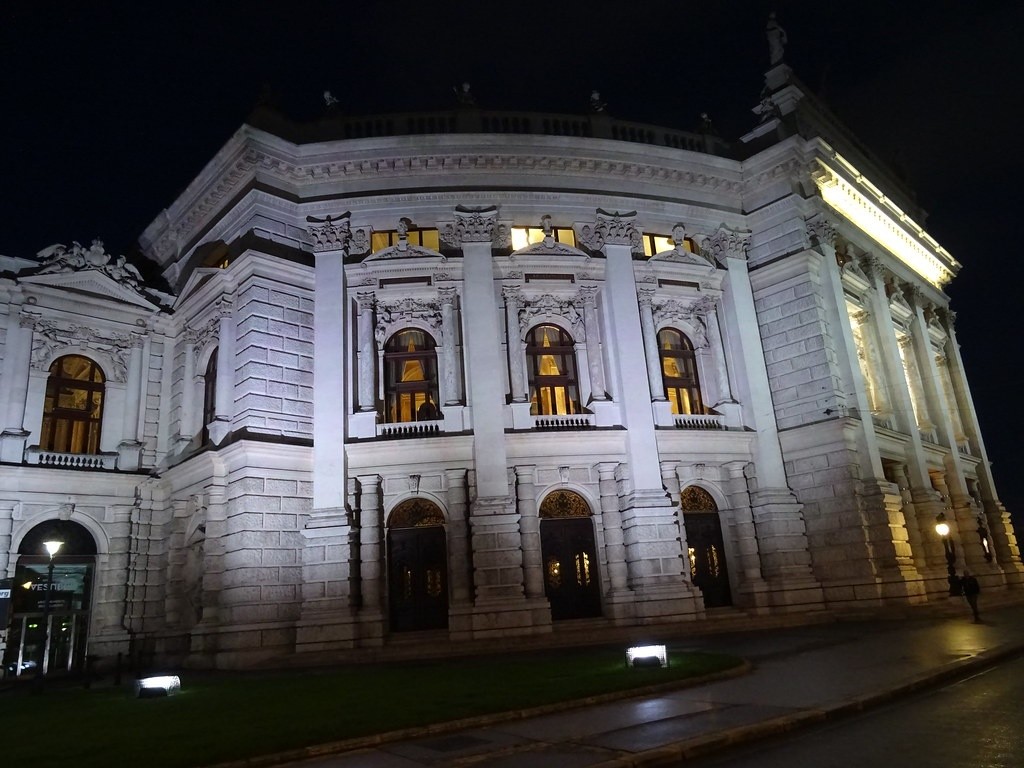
[935, 511, 963, 597]
[33, 539, 65, 679]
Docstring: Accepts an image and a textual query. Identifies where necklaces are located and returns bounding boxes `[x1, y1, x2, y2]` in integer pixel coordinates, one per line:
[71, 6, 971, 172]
[33, 247, 85, 275]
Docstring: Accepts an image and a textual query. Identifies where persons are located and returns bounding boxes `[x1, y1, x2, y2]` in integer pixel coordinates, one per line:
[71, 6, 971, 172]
[31, 329, 68, 362]
[324, 91, 345, 116]
[694, 112, 720, 137]
[765, 10, 787, 64]
[448, 82, 486, 111]
[108, 255, 142, 291]
[590, 90, 608, 113]
[98, 345, 126, 383]
[959, 570, 980, 622]
